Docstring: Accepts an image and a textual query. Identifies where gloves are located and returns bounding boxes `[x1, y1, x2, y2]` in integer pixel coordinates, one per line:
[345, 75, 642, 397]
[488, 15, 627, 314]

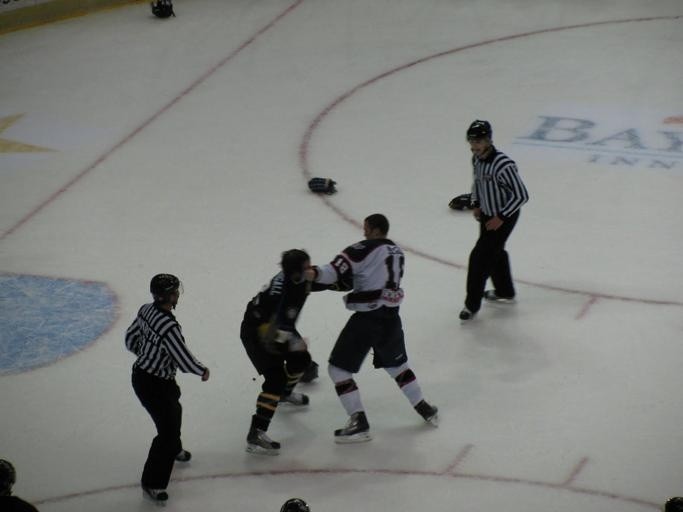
[448, 192, 476, 211]
[308, 176, 338, 195]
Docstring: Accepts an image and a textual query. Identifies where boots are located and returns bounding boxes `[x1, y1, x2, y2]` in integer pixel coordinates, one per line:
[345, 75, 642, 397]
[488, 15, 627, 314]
[279, 390, 309, 406]
[333, 409, 371, 437]
[413, 398, 439, 424]
[246, 414, 280, 450]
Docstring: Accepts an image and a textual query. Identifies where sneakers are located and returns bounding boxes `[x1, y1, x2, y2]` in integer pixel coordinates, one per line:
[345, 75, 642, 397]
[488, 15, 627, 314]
[485, 287, 514, 300]
[458, 306, 473, 320]
[176, 449, 192, 462]
[145, 487, 168, 501]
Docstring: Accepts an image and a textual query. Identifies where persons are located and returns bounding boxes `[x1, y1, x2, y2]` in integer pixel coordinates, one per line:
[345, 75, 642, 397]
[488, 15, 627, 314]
[303, 214, 437, 437]
[125, 274, 209, 500]
[240, 249, 354, 449]
[460, 121, 529, 320]
[0, 459, 38, 512]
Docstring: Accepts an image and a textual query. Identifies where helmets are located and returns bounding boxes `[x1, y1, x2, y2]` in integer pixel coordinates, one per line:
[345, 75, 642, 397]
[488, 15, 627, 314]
[150, 0, 176, 20]
[149, 273, 185, 302]
[465, 119, 494, 144]
